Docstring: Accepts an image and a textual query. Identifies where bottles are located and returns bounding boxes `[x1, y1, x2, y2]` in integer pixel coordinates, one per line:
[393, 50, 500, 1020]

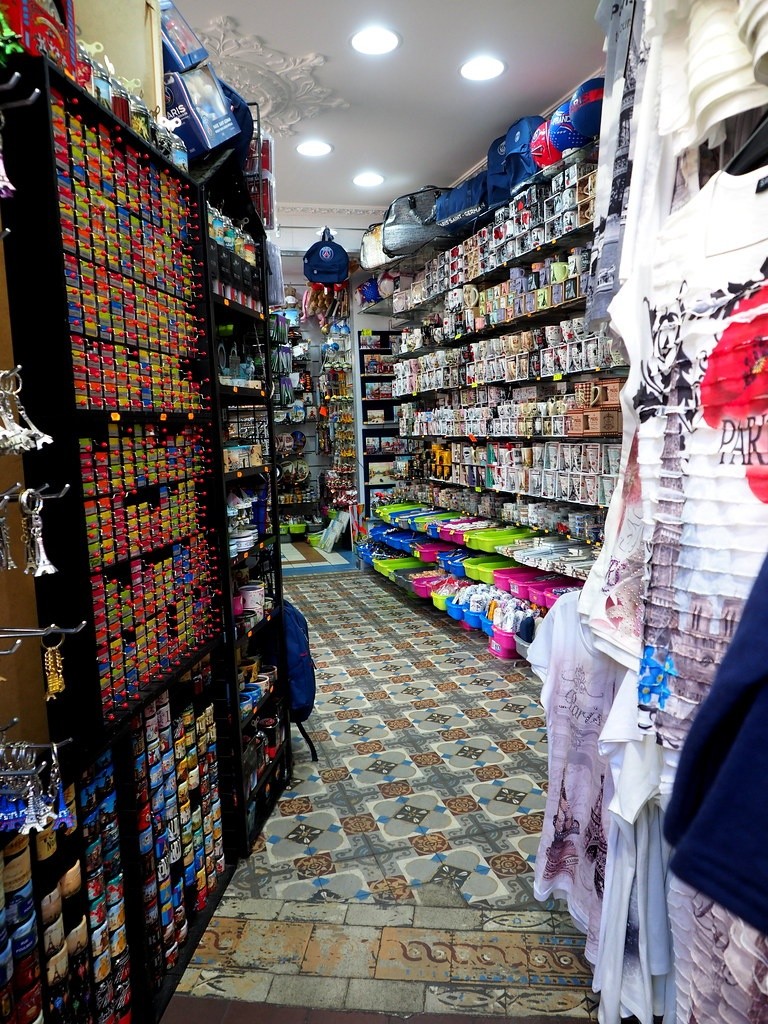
[77, 41, 188, 175]
[244, 234, 256, 266]
[222, 216, 235, 253]
[206, 201, 214, 240]
[234, 227, 245, 259]
[213, 208, 224, 246]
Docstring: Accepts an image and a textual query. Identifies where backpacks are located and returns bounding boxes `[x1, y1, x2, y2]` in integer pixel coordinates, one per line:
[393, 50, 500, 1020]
[501, 115, 547, 196]
[303, 228, 350, 284]
[487, 133, 512, 208]
[247, 594, 316, 722]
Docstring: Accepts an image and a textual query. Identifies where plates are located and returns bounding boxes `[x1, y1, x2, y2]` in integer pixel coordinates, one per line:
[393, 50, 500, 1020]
[274, 406, 287, 422]
[293, 460, 309, 482]
[275, 436, 280, 453]
[291, 431, 306, 452]
[276, 463, 283, 483]
[280, 461, 296, 483]
[277, 433, 294, 454]
[288, 405, 305, 422]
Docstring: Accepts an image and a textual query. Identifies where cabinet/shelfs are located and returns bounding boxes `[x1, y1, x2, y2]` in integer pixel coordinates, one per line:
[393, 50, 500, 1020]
[351, 137, 630, 662]
[1, 52, 362, 1024]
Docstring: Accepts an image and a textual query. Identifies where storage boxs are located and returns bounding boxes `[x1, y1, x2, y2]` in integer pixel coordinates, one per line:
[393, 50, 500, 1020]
[0, 0, 243, 159]
[567, 377, 626, 436]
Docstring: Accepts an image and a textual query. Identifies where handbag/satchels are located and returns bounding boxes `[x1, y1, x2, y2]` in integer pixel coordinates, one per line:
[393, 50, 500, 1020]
[359, 222, 412, 273]
[436, 168, 488, 233]
[382, 185, 457, 258]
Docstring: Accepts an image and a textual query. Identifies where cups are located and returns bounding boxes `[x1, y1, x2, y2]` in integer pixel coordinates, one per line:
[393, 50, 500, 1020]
[507, 448, 532, 493]
[499, 449, 508, 486]
[602, 478, 613, 505]
[238, 655, 277, 721]
[462, 446, 486, 486]
[533, 448, 543, 494]
[560, 448, 581, 501]
[393, 171, 627, 437]
[233, 565, 274, 638]
[546, 445, 557, 497]
[607, 447, 620, 474]
[586, 446, 598, 504]
[223, 444, 262, 473]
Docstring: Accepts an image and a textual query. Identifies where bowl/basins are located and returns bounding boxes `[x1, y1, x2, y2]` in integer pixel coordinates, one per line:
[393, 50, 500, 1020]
[229, 525, 258, 557]
